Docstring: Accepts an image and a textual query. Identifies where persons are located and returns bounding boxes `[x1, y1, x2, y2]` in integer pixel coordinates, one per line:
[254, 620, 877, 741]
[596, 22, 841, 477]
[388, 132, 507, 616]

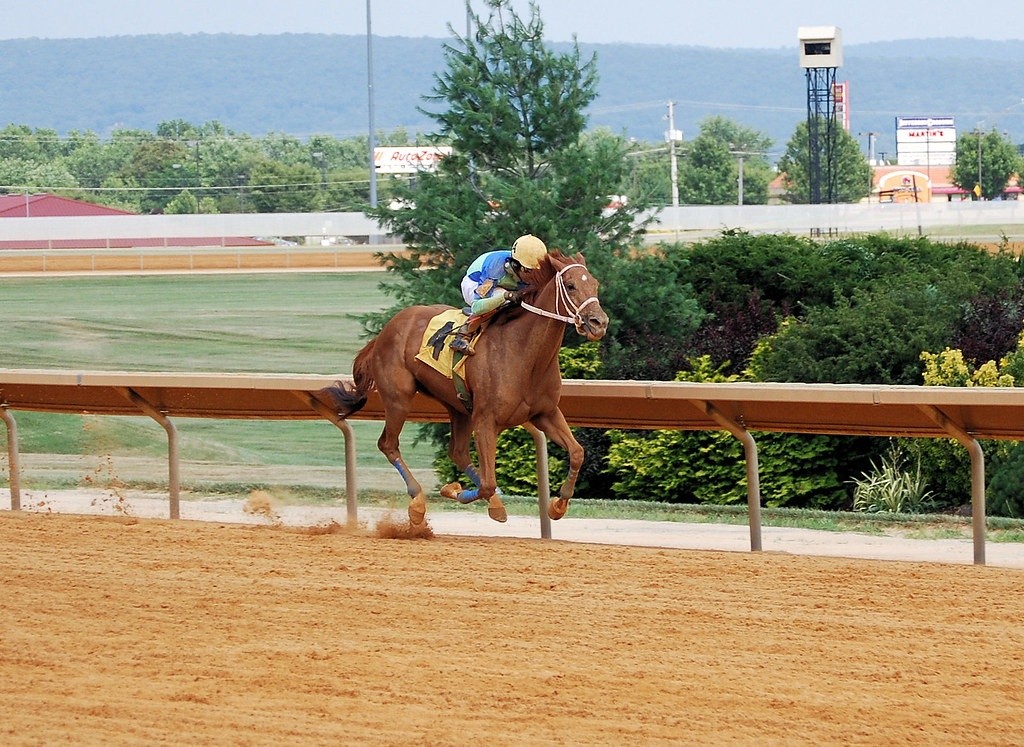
[449, 235, 547, 356]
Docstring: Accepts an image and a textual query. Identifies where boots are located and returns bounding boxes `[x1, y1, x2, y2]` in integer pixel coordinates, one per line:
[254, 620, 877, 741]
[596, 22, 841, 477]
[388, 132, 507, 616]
[449, 315, 476, 355]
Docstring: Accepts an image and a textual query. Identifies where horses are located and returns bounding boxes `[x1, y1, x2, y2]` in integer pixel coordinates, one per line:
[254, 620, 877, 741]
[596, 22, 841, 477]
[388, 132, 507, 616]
[312, 248, 610, 524]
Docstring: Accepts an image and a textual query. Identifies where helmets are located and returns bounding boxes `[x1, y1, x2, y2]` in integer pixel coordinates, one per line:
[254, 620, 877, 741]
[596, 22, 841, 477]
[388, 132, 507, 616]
[512, 234, 547, 269]
